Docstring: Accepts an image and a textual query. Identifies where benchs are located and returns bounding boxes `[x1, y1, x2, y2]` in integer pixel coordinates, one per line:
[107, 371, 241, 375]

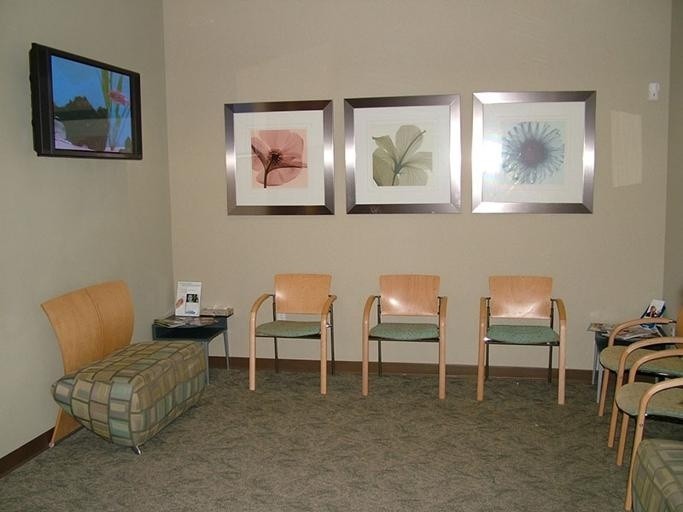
[40, 280, 208, 457]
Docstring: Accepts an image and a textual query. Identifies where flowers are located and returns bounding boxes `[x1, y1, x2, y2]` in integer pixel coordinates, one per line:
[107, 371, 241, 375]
[371, 125, 433, 187]
[502, 122, 565, 185]
[250, 129, 306, 189]
[100, 70, 130, 150]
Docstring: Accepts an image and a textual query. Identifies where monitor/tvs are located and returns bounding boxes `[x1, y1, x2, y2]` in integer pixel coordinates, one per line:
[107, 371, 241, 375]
[29, 43, 143, 160]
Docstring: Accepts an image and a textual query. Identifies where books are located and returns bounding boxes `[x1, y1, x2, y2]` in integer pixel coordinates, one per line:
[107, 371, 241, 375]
[174, 280, 202, 317]
[586, 298, 666, 341]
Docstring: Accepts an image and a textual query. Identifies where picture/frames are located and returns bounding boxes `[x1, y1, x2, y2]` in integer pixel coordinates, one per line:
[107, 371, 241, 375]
[224, 99, 335, 215]
[343, 94, 462, 214]
[470, 90, 597, 214]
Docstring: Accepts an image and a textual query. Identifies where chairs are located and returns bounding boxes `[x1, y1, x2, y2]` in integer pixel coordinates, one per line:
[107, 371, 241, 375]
[248, 273, 338, 395]
[361, 273, 448, 401]
[616, 348, 683, 512]
[475, 276, 567, 404]
[632, 439, 683, 512]
[598, 305, 683, 447]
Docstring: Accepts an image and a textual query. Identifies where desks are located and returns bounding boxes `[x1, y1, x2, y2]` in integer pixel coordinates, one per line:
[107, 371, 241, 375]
[591, 325, 677, 403]
[150, 312, 230, 385]
